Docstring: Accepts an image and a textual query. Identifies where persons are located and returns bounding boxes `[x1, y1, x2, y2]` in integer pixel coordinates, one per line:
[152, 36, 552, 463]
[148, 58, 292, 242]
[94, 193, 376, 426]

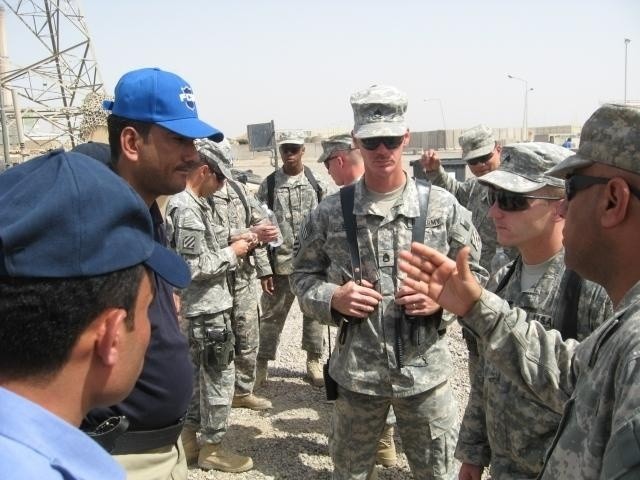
[454, 142, 614, 480]
[562, 138, 572, 148]
[289, 83, 490, 480]
[76, 68, 224, 480]
[164, 126, 366, 472]
[397, 102, 640, 480]
[422, 122, 518, 274]
[0, 146, 190, 478]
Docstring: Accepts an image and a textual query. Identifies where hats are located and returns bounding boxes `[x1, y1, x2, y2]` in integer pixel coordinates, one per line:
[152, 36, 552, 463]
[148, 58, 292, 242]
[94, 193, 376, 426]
[476, 142, 575, 194]
[279, 130, 305, 145]
[193, 137, 234, 179]
[219, 136, 233, 156]
[71, 141, 112, 167]
[103, 67, 223, 142]
[350, 84, 410, 141]
[317, 133, 357, 163]
[1, 147, 192, 288]
[458, 124, 496, 161]
[543, 103, 640, 180]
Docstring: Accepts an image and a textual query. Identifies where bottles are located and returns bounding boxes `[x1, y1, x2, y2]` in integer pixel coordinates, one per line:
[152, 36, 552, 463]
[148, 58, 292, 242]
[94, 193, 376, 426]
[259, 203, 284, 248]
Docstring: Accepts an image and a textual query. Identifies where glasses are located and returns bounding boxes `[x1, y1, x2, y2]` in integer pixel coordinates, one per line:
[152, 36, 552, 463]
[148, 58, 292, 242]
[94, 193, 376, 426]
[362, 137, 403, 151]
[205, 156, 224, 182]
[564, 173, 640, 200]
[487, 185, 559, 211]
[282, 145, 301, 153]
[465, 151, 494, 165]
[323, 157, 335, 169]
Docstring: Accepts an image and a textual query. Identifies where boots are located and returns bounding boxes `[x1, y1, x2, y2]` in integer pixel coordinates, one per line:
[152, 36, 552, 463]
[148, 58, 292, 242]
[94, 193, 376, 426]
[198, 441, 253, 472]
[232, 394, 272, 410]
[306, 353, 325, 386]
[253, 369, 267, 392]
[376, 425, 397, 466]
[182, 430, 200, 463]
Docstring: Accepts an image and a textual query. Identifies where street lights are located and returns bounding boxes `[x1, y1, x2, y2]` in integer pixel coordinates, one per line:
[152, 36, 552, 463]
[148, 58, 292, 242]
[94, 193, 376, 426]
[422, 97, 450, 150]
[508, 73, 535, 142]
[623, 37, 631, 104]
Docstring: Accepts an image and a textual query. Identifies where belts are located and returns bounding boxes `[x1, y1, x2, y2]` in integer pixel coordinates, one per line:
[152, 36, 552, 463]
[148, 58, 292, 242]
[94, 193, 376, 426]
[89, 414, 186, 455]
